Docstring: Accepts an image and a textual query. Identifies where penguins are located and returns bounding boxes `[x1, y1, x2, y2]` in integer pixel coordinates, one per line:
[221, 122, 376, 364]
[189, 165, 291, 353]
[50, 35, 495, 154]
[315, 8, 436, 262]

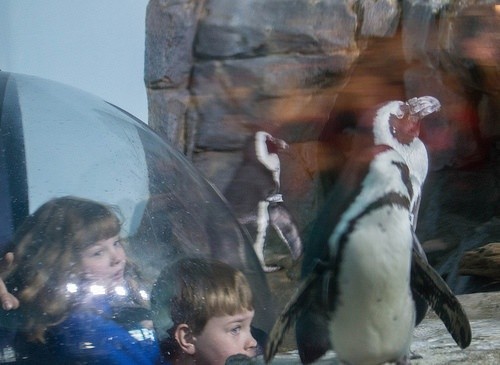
[222, 131, 304, 274]
[263, 92, 472, 365]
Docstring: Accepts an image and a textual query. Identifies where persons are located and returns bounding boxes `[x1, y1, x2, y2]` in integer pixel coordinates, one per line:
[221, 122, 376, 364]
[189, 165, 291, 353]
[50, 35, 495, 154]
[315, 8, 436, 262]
[149, 256, 259, 365]
[0, 194, 167, 365]
[0, 251, 21, 311]
